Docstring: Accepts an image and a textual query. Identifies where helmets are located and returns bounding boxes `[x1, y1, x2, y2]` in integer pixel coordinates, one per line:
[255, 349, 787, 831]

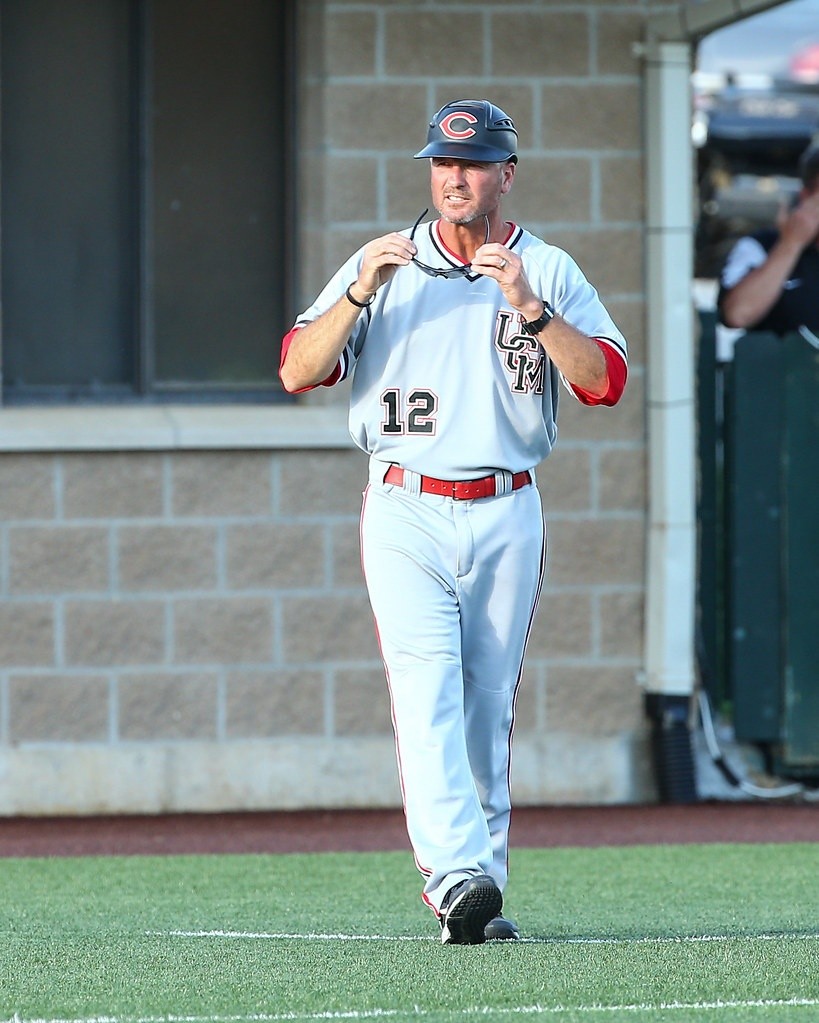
[413, 99, 518, 166]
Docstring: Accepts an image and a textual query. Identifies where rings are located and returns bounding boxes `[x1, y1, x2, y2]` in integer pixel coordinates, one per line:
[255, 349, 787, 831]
[499, 259, 507, 268]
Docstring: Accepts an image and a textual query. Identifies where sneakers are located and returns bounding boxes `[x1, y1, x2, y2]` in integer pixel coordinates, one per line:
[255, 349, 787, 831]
[441, 875, 503, 944]
[484, 912, 520, 940]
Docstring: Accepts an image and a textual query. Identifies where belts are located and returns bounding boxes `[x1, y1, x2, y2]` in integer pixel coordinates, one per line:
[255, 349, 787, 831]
[384, 465, 532, 501]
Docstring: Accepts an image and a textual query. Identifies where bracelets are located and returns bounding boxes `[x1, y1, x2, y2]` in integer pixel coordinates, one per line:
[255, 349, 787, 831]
[521, 301, 555, 336]
[346, 281, 376, 307]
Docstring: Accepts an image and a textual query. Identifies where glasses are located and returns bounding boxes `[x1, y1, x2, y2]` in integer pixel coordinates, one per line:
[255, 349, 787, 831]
[405, 208, 490, 278]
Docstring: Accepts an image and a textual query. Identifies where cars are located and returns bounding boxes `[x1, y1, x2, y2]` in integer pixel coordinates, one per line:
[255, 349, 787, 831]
[690, 1, 818, 192]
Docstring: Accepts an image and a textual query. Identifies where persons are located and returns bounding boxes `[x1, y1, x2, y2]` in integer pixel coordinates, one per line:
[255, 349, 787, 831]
[715, 135, 819, 334]
[279, 98, 629, 948]
